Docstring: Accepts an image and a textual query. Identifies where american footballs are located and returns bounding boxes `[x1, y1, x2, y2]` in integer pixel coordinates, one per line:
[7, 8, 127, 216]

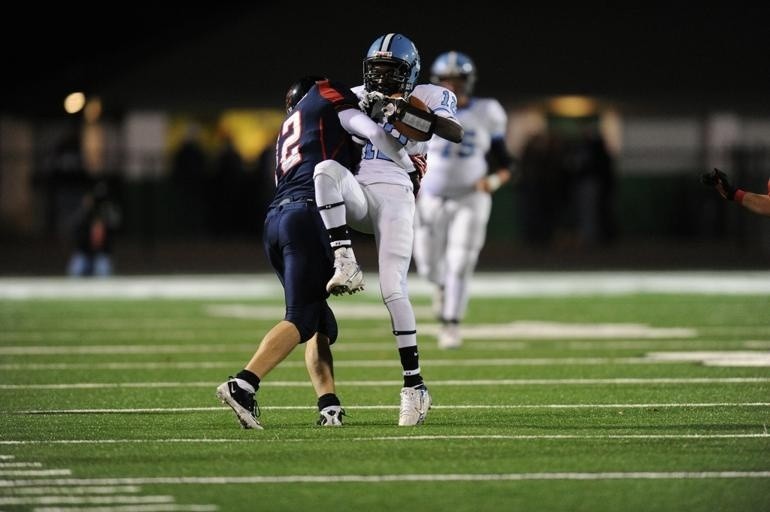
[387, 93, 433, 141]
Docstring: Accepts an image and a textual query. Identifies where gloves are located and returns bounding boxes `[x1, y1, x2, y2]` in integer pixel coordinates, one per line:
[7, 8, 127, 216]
[358, 91, 404, 127]
[408, 153, 429, 198]
[703, 167, 738, 203]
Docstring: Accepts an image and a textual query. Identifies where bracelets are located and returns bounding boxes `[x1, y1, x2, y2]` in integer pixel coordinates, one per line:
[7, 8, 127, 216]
[487, 173, 502, 192]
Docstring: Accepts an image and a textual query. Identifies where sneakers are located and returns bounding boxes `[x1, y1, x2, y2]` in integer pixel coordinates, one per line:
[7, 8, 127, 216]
[398, 387, 432, 427]
[325, 258, 365, 296]
[216, 379, 263, 430]
[317, 405, 342, 427]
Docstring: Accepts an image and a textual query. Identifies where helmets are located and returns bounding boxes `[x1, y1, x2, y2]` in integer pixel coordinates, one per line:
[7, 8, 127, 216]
[366, 32, 420, 84]
[285, 74, 321, 112]
[429, 49, 477, 92]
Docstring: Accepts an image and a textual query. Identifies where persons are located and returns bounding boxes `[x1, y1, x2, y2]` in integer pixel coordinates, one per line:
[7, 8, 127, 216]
[413, 51, 521, 350]
[312, 33, 464, 427]
[67, 196, 122, 276]
[216, 79, 427, 430]
[703, 168, 770, 216]
